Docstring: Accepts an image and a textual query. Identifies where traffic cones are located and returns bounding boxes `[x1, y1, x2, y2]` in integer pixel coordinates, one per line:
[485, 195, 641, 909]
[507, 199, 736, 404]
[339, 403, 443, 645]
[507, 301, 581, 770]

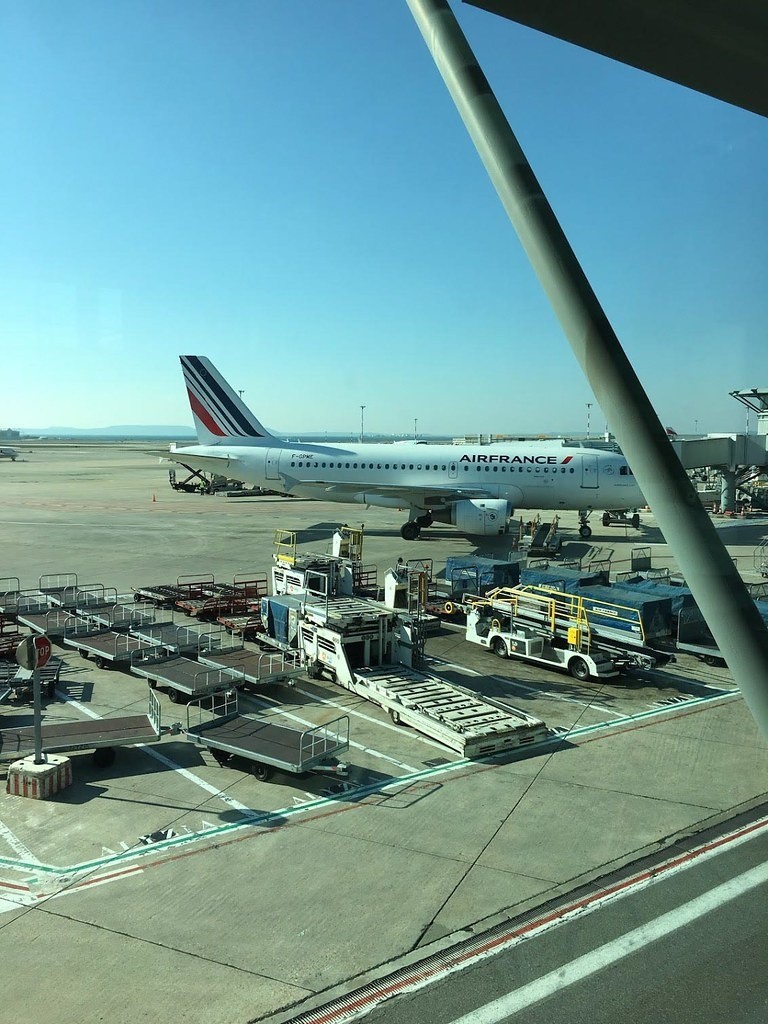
[713, 503, 718, 513]
[741, 507, 746, 516]
[749, 505, 753, 511]
[152, 493, 155, 501]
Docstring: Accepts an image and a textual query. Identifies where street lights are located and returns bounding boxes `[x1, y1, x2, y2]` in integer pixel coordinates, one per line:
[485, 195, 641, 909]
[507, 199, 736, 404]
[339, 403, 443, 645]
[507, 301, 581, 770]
[584, 402, 594, 439]
[413, 418, 419, 444]
[359, 405, 367, 444]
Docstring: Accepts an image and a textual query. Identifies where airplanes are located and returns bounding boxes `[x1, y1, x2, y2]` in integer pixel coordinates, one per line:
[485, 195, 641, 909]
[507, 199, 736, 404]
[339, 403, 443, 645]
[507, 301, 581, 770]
[0, 447, 19, 461]
[140, 355, 647, 540]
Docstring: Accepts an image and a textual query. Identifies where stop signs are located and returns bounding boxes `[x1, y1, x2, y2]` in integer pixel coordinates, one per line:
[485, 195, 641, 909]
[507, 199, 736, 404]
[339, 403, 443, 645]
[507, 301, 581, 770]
[33, 632, 52, 669]
